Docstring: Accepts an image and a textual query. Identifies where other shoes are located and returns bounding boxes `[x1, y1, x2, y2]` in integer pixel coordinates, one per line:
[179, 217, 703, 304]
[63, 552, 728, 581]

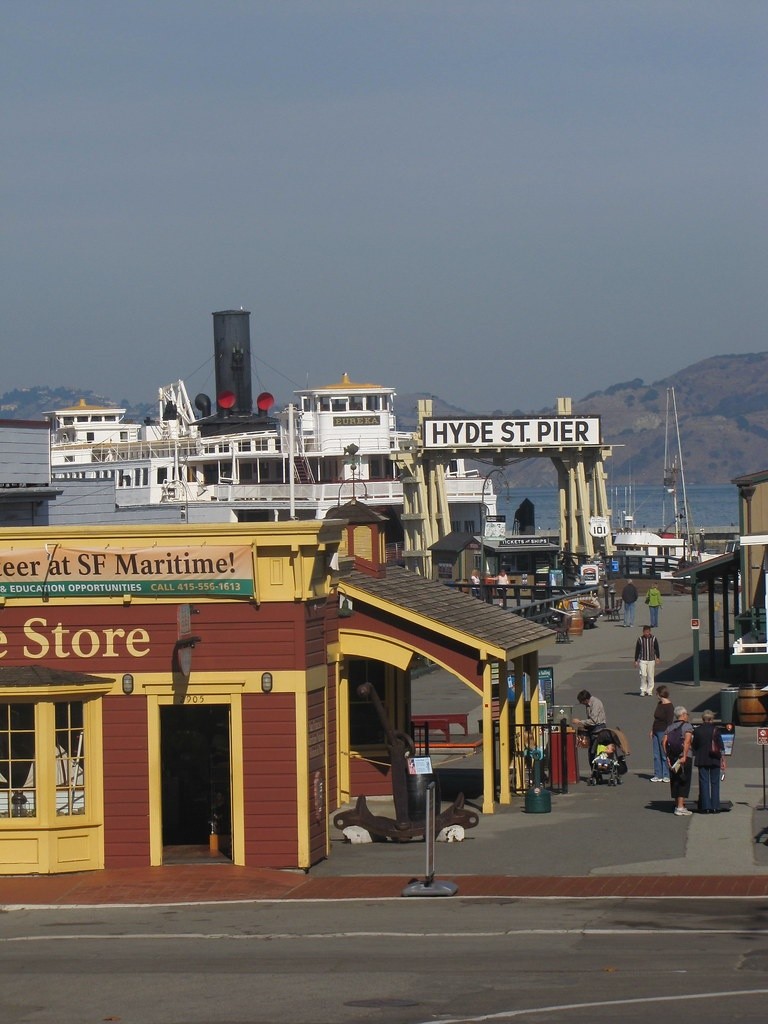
[650, 777, 662, 782]
[640, 692, 645, 696]
[674, 807, 692, 816]
[662, 777, 670, 783]
[695, 809, 709, 814]
[710, 808, 717, 814]
[623, 624, 626, 626]
[648, 691, 652, 696]
[598, 765, 606, 770]
[630, 625, 633, 627]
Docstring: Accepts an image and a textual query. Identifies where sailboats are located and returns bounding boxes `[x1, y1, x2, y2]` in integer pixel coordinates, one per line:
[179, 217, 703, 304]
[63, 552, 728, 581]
[611, 387, 742, 586]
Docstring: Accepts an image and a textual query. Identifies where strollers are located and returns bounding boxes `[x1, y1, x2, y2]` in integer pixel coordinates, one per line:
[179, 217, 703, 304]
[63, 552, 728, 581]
[586, 727, 624, 786]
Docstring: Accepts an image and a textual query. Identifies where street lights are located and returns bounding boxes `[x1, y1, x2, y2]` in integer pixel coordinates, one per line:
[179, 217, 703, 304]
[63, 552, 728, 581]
[481, 469, 509, 600]
[338, 477, 368, 507]
[161, 479, 189, 523]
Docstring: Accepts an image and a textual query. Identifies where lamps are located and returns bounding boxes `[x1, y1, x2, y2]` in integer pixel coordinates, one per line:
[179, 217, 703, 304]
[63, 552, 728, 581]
[313, 586, 353, 618]
[172, 636, 202, 673]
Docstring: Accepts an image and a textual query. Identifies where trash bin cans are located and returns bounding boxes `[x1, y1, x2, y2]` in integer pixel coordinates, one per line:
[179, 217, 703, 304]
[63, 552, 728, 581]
[719, 687, 739, 724]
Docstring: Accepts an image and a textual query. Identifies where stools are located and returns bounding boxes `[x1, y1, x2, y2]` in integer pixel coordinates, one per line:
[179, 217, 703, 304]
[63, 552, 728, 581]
[556, 628, 569, 643]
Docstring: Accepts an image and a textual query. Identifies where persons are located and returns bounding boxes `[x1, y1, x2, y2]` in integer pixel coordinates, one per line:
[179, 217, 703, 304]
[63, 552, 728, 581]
[488, 570, 509, 606]
[573, 690, 606, 780]
[592, 743, 616, 769]
[634, 626, 660, 695]
[650, 685, 674, 782]
[691, 710, 726, 813]
[662, 707, 692, 815]
[471, 569, 481, 598]
[623, 579, 638, 627]
[646, 582, 663, 627]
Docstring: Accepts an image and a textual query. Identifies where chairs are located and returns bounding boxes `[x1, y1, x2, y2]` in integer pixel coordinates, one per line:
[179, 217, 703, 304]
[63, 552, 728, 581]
[607, 599, 623, 622]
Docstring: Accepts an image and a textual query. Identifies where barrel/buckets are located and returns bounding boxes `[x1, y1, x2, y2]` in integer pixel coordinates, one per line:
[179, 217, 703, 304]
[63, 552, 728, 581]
[563, 608, 584, 635]
[406, 772, 442, 822]
[737, 683, 768, 727]
[454, 579, 470, 594]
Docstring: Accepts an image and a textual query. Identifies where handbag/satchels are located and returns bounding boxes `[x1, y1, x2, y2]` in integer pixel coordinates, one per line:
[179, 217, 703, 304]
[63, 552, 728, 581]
[617, 759, 627, 774]
[666, 722, 686, 762]
[576, 728, 589, 748]
[645, 589, 650, 604]
[710, 727, 720, 758]
